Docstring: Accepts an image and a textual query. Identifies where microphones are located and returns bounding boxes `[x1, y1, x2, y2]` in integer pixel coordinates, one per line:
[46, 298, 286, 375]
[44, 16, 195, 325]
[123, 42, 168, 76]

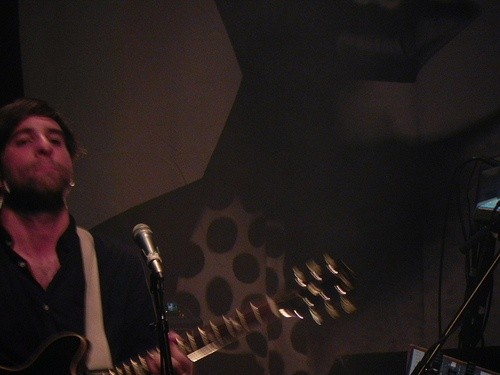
[132, 223, 164, 281]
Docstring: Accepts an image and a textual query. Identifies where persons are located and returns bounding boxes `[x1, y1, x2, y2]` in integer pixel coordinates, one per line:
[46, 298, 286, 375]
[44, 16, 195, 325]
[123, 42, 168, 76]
[0, 98, 193, 375]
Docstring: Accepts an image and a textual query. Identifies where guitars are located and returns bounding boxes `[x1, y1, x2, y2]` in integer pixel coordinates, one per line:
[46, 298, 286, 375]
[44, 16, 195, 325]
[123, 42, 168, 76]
[0, 252, 359, 375]
